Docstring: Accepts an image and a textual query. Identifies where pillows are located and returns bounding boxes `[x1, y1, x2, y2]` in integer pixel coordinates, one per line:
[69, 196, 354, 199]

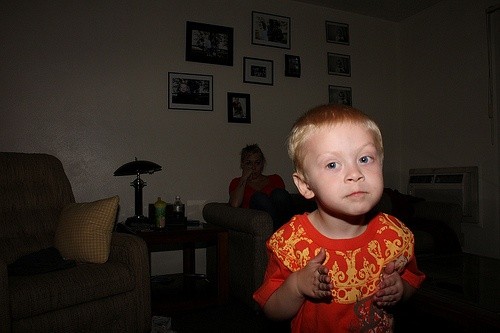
[55, 195, 120, 264]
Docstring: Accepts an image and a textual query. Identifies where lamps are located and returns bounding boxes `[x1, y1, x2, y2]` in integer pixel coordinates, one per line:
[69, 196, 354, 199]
[113, 156, 162, 224]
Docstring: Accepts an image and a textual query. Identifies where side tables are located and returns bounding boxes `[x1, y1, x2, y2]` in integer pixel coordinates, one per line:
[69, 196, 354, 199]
[117, 223, 230, 315]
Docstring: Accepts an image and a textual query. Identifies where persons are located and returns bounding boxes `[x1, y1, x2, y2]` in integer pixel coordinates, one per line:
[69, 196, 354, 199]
[228, 144, 300, 232]
[201, 30, 223, 59]
[337, 91, 347, 103]
[175, 83, 197, 104]
[252, 104, 426, 333]
[233, 98, 244, 118]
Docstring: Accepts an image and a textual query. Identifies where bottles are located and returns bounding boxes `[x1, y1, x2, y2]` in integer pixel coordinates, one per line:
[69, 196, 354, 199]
[173, 195, 184, 225]
[154, 197, 167, 231]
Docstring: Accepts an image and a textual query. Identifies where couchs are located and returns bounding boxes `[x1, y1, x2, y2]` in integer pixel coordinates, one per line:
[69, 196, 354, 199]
[202, 193, 481, 316]
[0, 152, 153, 333]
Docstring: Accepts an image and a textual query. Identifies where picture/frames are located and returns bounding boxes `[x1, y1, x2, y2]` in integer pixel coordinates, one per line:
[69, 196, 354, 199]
[285, 54, 301, 78]
[327, 52, 351, 77]
[328, 85, 352, 107]
[325, 20, 350, 46]
[227, 92, 251, 124]
[243, 56, 274, 86]
[251, 11, 291, 50]
[185, 21, 234, 66]
[168, 72, 214, 111]
